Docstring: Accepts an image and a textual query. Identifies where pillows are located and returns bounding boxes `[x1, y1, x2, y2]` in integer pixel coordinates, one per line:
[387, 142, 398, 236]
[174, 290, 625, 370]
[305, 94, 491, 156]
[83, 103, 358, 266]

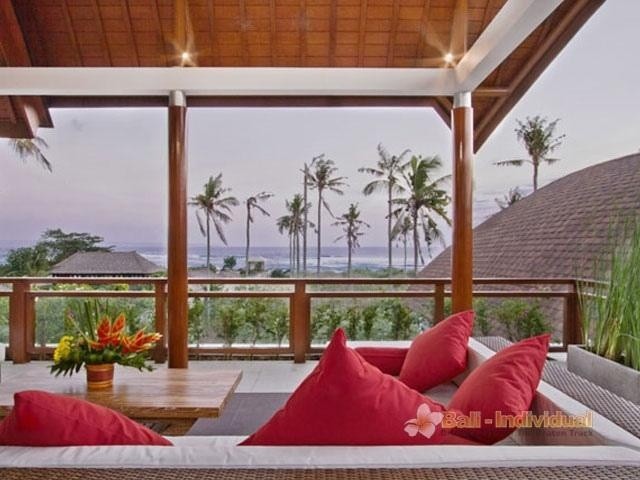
[446, 332, 551, 442]
[235, 328, 468, 445]
[400, 308, 473, 391]
[0, 389, 174, 446]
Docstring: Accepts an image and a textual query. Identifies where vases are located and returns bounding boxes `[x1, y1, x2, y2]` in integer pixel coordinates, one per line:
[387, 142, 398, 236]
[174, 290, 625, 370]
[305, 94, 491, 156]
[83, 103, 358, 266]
[85, 363, 115, 390]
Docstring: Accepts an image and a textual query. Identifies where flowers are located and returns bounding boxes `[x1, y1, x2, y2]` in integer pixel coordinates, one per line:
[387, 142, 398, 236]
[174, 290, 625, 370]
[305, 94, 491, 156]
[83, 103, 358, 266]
[51, 301, 161, 361]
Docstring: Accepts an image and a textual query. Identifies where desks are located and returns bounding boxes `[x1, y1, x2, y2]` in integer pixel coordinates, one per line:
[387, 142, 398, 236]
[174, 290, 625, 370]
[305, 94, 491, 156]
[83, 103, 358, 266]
[0, 361, 244, 435]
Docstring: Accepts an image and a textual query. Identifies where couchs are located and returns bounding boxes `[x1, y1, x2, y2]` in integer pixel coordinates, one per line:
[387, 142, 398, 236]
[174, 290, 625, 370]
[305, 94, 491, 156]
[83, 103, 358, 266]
[0, 336, 640, 480]
